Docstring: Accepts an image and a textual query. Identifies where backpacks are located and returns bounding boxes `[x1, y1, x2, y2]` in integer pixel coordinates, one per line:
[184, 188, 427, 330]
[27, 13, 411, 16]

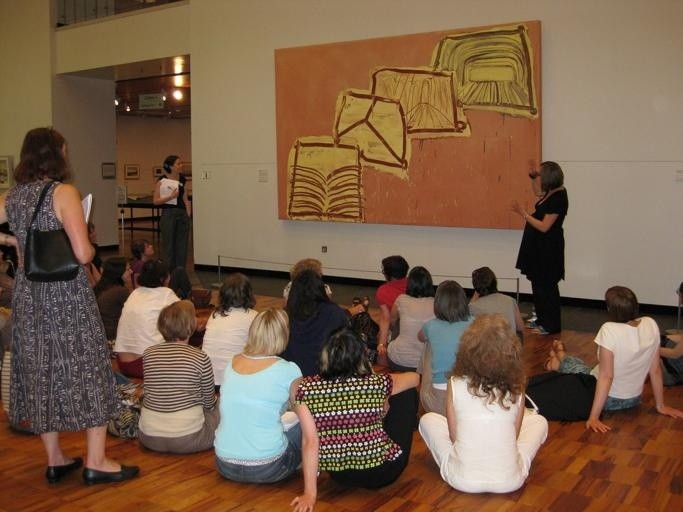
[524, 369, 596, 422]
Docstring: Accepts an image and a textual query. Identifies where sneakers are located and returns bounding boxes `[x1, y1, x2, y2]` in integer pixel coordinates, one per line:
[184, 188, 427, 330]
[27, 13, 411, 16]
[532, 325, 549, 335]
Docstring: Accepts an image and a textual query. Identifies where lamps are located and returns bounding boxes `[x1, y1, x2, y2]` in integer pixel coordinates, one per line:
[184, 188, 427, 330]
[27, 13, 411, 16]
[115, 96, 121, 106]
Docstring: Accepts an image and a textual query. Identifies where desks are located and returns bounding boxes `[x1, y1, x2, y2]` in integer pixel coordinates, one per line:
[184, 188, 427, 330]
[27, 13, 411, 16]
[117, 195, 162, 237]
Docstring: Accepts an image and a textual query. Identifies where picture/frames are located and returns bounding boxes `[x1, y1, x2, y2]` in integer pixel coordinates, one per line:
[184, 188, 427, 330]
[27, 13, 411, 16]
[0, 155, 13, 189]
[124, 164, 141, 180]
[153, 166, 164, 176]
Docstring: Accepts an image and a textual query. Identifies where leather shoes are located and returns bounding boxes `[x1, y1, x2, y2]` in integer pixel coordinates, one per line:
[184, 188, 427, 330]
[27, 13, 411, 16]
[45, 456, 82, 485]
[81, 463, 138, 486]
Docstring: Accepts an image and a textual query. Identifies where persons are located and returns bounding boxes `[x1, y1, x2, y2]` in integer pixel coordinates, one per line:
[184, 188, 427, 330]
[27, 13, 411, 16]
[6, 127, 139, 486]
[545, 282, 683, 433]
[83, 224, 548, 510]
[1, 220, 21, 292]
[153, 154, 192, 273]
[510, 162, 569, 335]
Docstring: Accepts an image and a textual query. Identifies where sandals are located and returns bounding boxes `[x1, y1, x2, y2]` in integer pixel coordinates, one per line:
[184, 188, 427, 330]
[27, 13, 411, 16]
[544, 348, 555, 371]
[360, 296, 370, 312]
[552, 339, 567, 355]
[351, 296, 360, 307]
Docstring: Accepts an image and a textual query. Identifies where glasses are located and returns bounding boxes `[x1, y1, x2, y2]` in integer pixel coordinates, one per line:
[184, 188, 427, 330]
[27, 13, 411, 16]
[534, 172, 540, 177]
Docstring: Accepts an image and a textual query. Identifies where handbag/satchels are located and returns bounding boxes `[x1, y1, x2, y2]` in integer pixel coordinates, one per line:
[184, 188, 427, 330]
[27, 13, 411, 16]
[189, 290, 211, 306]
[107, 383, 145, 439]
[23, 228, 79, 282]
[349, 311, 378, 349]
